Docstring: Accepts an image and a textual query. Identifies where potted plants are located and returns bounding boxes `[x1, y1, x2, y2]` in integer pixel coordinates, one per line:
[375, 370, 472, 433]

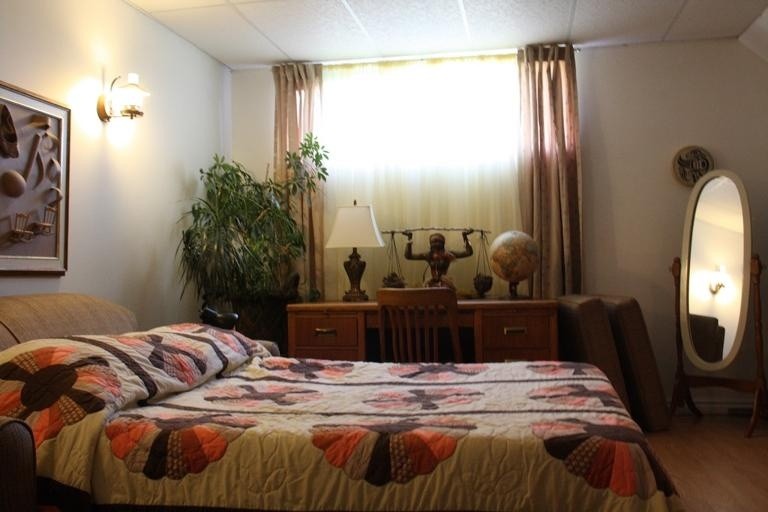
[171, 131, 329, 339]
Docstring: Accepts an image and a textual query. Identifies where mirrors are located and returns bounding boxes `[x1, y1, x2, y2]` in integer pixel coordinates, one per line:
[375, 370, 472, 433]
[664, 170, 768, 436]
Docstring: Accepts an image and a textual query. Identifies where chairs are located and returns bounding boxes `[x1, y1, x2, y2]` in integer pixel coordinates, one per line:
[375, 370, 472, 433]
[377, 286, 462, 363]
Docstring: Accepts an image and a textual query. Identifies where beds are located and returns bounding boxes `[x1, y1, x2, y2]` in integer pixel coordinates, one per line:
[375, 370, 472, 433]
[0, 293, 684, 512]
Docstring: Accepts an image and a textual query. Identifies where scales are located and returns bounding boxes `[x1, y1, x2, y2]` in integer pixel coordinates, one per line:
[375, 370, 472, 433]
[381, 226, 494, 294]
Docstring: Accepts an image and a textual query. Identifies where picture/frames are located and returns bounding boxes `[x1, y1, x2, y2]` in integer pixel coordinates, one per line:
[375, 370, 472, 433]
[0, 80, 72, 277]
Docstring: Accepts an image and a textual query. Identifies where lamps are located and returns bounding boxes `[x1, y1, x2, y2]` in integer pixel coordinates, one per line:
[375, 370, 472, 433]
[325, 199, 385, 302]
[97, 73, 153, 122]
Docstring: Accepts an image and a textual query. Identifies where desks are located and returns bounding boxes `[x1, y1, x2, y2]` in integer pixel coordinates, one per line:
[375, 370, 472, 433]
[287, 296, 560, 361]
